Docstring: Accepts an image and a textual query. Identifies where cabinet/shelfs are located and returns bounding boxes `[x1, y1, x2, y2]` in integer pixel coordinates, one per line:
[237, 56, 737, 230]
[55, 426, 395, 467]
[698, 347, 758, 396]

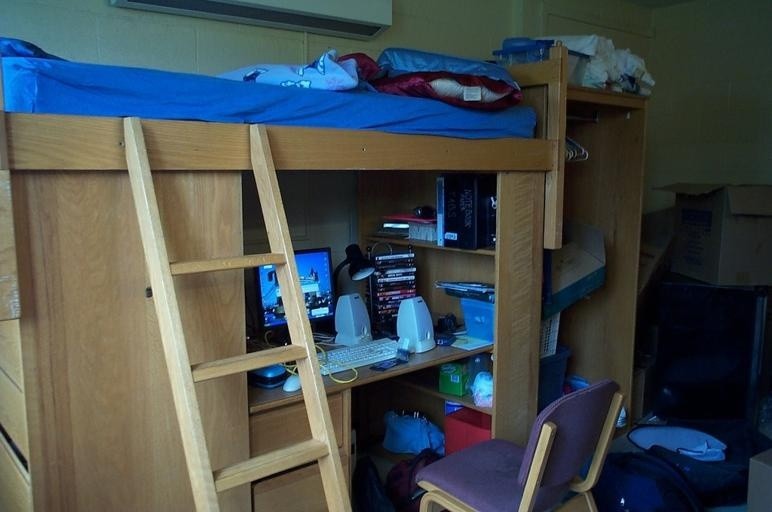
[0, 178, 249, 512]
[358, 174, 543, 459]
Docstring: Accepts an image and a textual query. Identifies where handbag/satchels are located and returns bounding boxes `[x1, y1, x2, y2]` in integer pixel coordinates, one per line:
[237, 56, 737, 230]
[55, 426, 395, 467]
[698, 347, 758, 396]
[382, 411, 445, 456]
[387, 448, 439, 512]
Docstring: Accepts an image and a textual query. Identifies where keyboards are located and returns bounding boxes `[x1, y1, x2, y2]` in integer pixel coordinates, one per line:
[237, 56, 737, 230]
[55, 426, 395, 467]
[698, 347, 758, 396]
[315, 338, 402, 376]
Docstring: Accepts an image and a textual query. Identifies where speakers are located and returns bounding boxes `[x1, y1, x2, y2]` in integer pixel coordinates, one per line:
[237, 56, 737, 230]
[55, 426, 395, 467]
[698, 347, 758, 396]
[334, 293, 373, 347]
[397, 296, 436, 354]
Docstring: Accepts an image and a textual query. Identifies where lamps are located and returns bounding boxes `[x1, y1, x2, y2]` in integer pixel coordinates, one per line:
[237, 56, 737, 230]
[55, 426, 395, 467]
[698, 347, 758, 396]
[333, 242, 373, 294]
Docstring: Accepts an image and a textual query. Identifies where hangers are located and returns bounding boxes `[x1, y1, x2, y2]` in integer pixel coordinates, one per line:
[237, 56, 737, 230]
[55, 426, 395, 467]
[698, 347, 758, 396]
[566, 134, 590, 163]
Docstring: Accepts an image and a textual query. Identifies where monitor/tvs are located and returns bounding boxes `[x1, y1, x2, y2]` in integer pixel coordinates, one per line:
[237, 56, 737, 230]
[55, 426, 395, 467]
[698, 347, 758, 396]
[256, 247, 337, 345]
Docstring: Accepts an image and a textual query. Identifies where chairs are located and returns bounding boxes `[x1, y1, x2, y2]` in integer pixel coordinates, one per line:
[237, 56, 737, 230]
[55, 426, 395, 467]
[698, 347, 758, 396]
[414, 380, 626, 512]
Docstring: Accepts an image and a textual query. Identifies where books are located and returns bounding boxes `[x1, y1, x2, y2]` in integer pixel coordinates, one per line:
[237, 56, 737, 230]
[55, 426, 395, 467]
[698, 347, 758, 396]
[374, 253, 416, 323]
[434, 280, 495, 303]
[451, 335, 492, 352]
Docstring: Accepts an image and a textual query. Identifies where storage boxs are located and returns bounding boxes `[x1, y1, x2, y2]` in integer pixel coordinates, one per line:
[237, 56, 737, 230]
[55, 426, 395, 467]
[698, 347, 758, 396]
[649, 172, 772, 288]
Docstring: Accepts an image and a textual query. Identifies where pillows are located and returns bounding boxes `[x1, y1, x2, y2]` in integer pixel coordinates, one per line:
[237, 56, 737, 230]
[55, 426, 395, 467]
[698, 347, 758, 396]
[377, 46, 523, 112]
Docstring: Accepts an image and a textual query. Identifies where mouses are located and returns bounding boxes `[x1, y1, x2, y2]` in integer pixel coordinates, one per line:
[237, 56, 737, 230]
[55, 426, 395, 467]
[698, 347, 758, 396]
[283, 374, 302, 391]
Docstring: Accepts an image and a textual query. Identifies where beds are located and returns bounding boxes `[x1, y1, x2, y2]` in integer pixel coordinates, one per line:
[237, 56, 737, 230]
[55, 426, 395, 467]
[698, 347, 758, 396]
[2, 29, 547, 193]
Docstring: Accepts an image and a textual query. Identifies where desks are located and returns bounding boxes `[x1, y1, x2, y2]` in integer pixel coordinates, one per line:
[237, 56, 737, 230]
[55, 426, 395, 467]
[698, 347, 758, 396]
[250, 331, 495, 512]
